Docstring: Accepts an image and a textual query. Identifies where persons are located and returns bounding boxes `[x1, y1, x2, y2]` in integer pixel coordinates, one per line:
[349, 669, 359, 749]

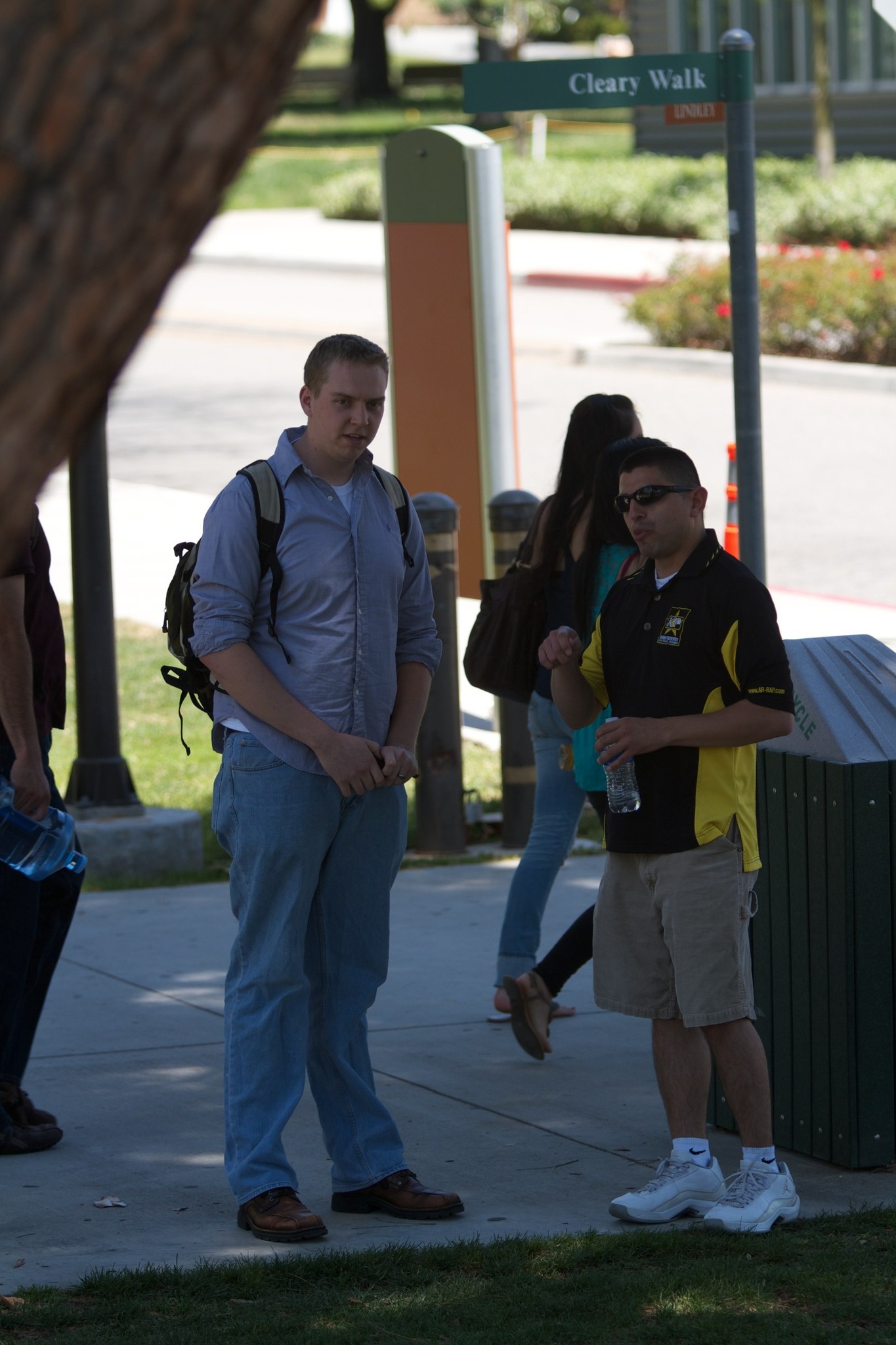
[507, 436, 668, 1059]
[538, 447, 800, 1237]
[488, 394, 645, 1024]
[185, 336, 465, 1244]
[0, 504, 86, 1156]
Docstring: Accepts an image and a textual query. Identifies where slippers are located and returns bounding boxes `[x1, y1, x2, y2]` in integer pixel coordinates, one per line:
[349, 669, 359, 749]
[487, 1002, 575, 1022]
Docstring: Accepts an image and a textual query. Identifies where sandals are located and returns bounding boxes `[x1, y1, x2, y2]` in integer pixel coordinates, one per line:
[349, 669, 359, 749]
[503, 971, 552, 1061]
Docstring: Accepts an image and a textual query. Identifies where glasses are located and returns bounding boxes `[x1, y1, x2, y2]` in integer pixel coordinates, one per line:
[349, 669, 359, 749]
[614, 485, 695, 513]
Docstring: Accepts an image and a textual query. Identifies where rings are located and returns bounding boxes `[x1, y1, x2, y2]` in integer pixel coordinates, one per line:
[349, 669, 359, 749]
[397, 774, 405, 779]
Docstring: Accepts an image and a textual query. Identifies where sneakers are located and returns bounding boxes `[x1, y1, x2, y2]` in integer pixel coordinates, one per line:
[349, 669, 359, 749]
[703, 1150, 801, 1235]
[608, 1150, 727, 1224]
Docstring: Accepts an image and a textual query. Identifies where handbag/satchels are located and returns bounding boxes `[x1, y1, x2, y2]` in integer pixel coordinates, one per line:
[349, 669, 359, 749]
[464, 493, 555, 705]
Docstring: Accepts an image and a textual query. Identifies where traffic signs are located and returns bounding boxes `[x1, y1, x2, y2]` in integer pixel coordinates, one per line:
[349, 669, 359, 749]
[463, 52, 725, 111]
[663, 102, 725, 124]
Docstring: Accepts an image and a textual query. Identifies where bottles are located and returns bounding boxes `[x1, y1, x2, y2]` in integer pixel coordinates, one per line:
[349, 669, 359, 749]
[600, 717, 641, 813]
[0, 779, 89, 882]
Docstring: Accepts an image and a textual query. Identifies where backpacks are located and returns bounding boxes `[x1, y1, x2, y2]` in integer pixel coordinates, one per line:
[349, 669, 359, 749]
[161, 459, 414, 756]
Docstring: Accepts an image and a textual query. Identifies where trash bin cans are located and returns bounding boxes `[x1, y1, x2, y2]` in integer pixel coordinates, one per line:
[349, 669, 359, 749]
[746, 632, 896, 1175]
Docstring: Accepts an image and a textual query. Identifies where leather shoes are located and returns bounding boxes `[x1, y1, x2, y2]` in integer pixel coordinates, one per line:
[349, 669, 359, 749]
[331, 1169, 464, 1219]
[237, 1186, 329, 1242]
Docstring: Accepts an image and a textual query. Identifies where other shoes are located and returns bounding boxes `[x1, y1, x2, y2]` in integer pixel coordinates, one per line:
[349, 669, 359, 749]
[0, 1079, 57, 1125]
[0, 1123, 63, 1154]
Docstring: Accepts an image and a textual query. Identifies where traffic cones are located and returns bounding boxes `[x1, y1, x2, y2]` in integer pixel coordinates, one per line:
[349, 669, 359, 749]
[724, 444, 739, 559]
